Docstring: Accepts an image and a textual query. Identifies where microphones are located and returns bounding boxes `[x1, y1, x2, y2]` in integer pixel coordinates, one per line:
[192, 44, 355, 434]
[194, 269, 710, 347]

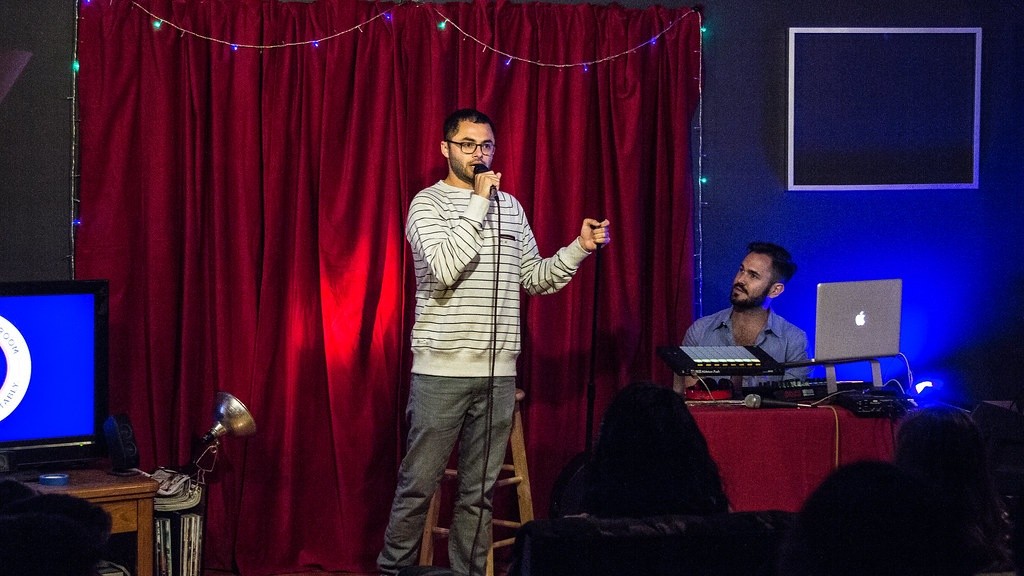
[745, 394, 816, 409]
[473, 164, 499, 203]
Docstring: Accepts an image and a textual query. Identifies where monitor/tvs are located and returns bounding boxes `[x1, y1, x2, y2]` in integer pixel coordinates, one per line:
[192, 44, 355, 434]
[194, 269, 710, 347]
[0, 279, 111, 471]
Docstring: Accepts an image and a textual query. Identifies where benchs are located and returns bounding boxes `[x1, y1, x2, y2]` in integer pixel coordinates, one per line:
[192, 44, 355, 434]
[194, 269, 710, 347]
[509, 510, 795, 576]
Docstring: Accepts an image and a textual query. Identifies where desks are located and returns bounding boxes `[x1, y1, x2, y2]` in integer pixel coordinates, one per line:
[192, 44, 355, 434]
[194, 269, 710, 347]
[683, 397, 918, 516]
[0, 455, 160, 576]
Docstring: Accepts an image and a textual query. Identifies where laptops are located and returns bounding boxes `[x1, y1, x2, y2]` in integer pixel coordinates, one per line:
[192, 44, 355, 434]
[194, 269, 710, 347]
[657, 346, 784, 376]
[780, 278, 903, 366]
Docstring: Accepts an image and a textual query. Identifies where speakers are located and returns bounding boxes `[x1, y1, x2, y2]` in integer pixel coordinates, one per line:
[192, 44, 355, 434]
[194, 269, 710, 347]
[104, 414, 140, 477]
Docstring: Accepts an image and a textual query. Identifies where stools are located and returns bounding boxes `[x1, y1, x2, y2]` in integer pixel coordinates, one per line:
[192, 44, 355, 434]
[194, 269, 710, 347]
[418, 386, 537, 576]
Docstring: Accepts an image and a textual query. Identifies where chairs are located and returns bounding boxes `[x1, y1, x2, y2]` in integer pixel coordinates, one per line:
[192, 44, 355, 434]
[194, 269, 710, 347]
[585, 383, 737, 517]
[0, 493, 133, 576]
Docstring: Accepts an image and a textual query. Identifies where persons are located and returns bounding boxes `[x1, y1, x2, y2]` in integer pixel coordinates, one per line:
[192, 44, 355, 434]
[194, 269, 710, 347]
[496, 381, 1024, 576]
[374, 106, 610, 574]
[669, 242, 815, 410]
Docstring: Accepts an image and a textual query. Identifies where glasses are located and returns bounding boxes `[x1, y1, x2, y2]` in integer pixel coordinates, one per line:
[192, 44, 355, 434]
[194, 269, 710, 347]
[446, 139, 496, 157]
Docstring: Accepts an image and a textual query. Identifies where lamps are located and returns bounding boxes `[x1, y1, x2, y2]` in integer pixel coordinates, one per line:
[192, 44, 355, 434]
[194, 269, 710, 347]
[187, 391, 257, 489]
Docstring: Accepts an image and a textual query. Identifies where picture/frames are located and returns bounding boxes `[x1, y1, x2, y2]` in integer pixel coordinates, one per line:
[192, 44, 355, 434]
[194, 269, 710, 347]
[783, 24, 983, 192]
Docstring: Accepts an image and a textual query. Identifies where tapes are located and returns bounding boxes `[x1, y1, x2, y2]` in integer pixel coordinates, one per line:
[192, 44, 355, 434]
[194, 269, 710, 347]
[38, 473, 70, 486]
